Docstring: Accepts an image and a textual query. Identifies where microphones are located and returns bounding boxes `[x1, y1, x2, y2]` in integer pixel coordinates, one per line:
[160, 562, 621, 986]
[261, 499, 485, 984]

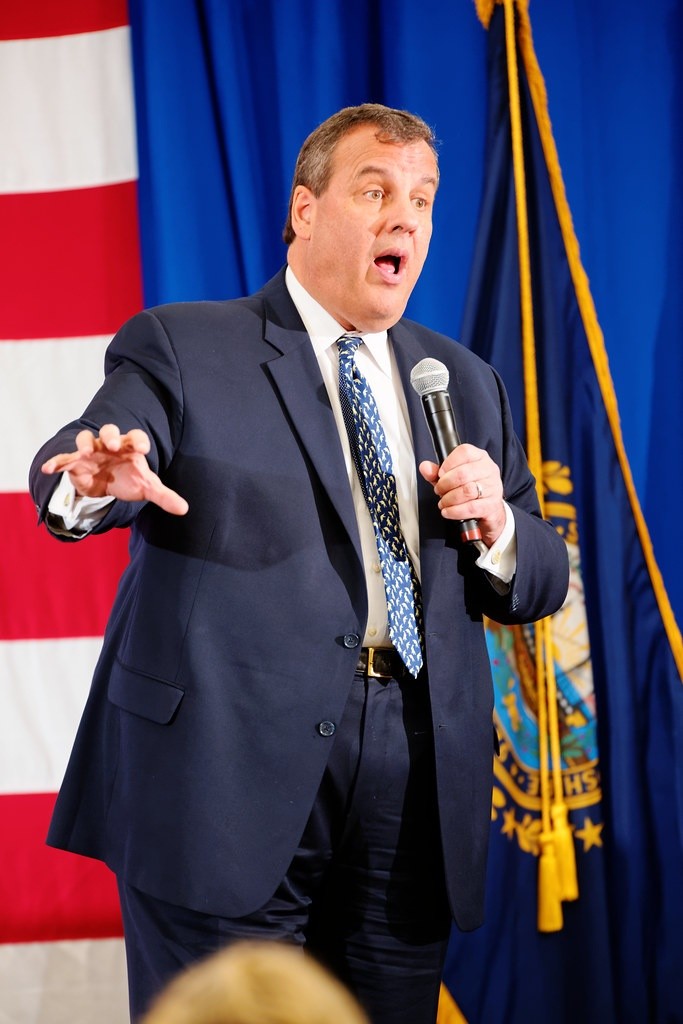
[410, 358, 482, 545]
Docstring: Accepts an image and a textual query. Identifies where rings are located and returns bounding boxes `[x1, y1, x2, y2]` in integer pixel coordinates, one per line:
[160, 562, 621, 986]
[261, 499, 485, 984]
[475, 481, 483, 499]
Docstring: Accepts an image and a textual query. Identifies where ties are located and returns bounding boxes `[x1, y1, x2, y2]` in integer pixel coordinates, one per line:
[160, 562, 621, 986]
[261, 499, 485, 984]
[337, 335, 427, 681]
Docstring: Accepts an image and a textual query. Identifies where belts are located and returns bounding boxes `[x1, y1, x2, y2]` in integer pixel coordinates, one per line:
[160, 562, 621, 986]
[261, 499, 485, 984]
[356, 644, 404, 679]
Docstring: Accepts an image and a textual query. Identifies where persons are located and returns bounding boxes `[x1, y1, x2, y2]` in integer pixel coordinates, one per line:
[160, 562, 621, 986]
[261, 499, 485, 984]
[29, 104, 570, 1024]
